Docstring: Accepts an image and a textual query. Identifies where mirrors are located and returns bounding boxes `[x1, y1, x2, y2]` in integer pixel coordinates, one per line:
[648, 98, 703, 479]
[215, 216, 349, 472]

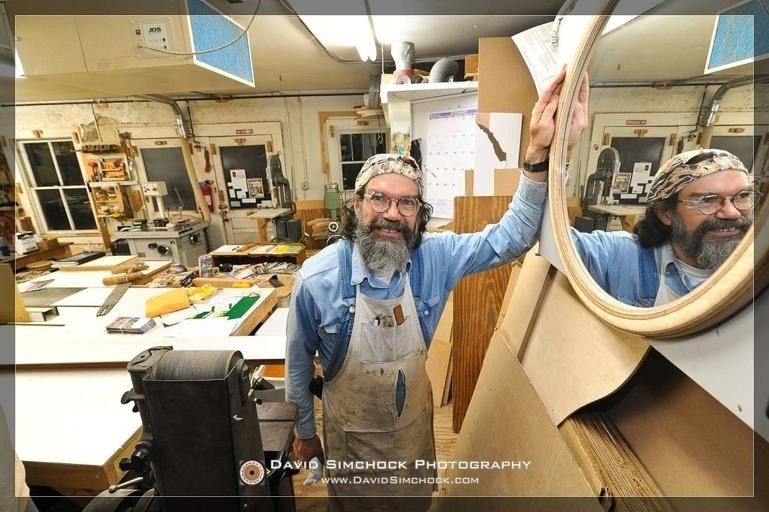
[544, 0, 769, 337]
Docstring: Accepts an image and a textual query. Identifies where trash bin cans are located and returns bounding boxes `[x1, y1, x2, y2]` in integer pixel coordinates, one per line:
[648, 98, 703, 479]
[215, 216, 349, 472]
[110, 238, 131, 256]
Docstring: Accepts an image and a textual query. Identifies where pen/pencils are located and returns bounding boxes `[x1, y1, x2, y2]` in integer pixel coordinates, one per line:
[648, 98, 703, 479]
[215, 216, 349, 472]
[1, 322, 65, 327]
[232, 246, 239, 251]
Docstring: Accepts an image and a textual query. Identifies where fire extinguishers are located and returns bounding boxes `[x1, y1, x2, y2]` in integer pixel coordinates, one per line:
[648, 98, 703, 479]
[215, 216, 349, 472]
[199, 179, 214, 213]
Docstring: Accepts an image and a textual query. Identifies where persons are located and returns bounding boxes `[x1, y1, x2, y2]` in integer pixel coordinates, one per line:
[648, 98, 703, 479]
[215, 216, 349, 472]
[284, 53, 570, 509]
[565, 71, 757, 307]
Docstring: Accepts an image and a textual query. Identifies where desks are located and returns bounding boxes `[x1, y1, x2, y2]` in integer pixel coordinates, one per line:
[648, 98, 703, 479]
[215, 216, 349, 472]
[584, 203, 650, 221]
[0, 263, 277, 491]
[0, 241, 74, 272]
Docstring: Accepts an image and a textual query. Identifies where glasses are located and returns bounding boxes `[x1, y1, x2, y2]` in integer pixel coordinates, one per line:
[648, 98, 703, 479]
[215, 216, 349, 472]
[358, 192, 424, 217]
[674, 184, 766, 217]
